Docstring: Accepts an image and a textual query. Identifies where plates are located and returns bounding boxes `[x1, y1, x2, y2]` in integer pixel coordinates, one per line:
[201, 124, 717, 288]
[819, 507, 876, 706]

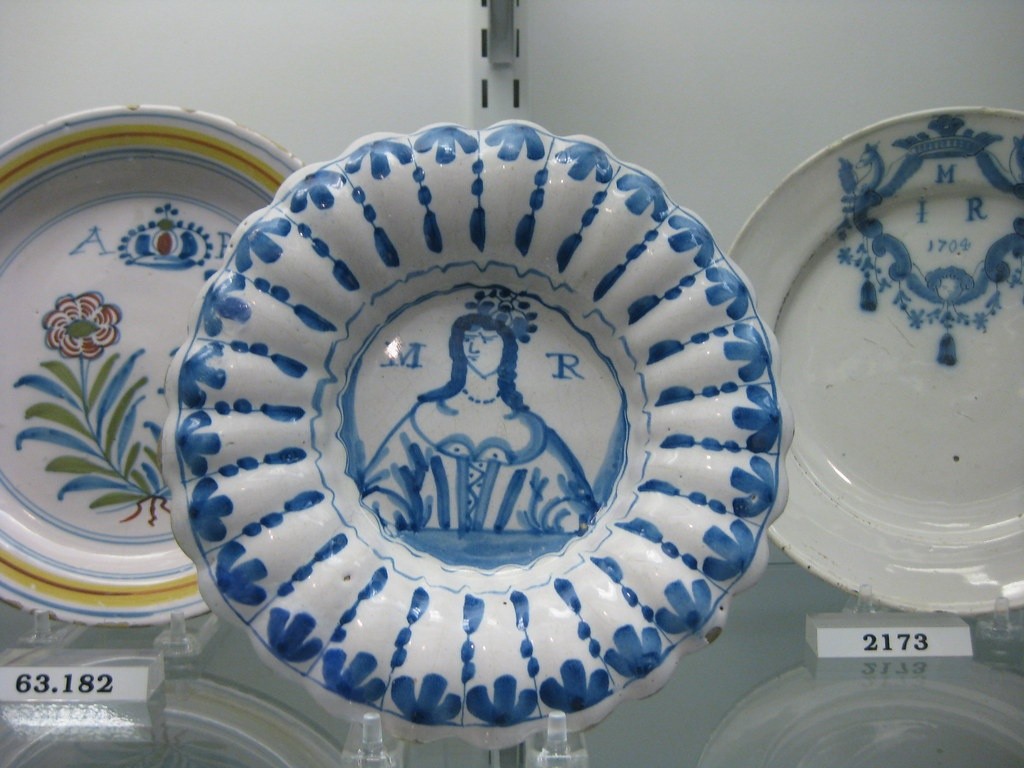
[727, 105, 1024, 616]
[0, 104, 302, 627]
[160, 120, 791, 750]
